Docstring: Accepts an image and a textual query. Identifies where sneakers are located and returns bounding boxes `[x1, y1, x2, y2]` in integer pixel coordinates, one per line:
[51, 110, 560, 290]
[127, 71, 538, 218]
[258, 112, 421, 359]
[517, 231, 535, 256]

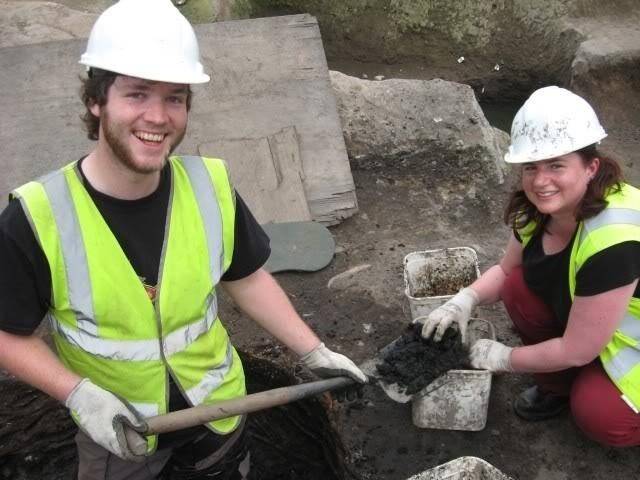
[515, 383, 567, 421]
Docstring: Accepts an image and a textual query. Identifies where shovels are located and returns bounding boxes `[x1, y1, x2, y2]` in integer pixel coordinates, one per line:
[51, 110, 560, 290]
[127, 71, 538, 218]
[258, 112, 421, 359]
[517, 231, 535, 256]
[121, 334, 452, 456]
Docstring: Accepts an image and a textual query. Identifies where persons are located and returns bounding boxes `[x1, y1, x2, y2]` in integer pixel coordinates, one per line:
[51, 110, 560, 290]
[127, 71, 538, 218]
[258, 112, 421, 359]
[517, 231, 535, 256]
[420, 83, 639, 446]
[0, 0, 369, 479]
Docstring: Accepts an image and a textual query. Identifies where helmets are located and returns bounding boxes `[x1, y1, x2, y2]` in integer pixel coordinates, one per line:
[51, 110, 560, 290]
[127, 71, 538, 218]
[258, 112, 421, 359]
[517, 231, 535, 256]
[504, 86, 608, 164]
[79, 0, 211, 85]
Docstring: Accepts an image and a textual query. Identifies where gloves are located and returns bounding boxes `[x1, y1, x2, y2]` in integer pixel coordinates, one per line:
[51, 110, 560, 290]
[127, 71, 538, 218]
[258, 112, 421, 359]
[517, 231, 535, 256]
[301, 341, 368, 402]
[421, 288, 479, 344]
[66, 378, 148, 461]
[469, 339, 515, 373]
